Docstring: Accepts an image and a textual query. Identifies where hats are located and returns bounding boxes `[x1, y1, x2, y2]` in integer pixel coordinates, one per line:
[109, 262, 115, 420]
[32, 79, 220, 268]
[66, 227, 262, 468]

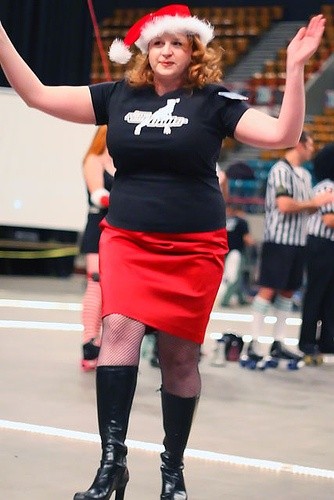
[109, 4, 215, 64]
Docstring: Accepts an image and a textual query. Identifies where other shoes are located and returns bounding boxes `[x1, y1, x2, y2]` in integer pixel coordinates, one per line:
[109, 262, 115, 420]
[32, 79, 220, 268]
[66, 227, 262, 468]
[152, 343, 160, 367]
[304, 354, 323, 366]
[81, 337, 100, 372]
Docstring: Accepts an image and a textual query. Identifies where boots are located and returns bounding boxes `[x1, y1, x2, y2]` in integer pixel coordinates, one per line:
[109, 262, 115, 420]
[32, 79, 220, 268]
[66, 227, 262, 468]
[159, 384, 201, 500]
[72, 365, 139, 500]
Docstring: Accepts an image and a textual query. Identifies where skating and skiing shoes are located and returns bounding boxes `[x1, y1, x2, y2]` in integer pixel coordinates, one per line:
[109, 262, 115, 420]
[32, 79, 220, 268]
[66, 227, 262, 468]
[266, 341, 302, 370]
[239, 338, 267, 370]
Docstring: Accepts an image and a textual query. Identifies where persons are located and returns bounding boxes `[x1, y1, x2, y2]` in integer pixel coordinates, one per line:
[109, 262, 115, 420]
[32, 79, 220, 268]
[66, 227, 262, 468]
[81, 125, 116, 371]
[0, 4, 327, 500]
[216, 131, 334, 370]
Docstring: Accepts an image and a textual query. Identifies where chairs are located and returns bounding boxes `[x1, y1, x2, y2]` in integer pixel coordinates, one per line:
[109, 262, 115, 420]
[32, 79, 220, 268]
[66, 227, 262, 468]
[91, 7, 334, 212]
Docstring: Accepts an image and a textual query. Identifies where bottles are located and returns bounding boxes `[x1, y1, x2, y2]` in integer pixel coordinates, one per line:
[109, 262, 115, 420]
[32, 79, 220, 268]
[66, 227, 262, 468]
[209, 339, 225, 366]
